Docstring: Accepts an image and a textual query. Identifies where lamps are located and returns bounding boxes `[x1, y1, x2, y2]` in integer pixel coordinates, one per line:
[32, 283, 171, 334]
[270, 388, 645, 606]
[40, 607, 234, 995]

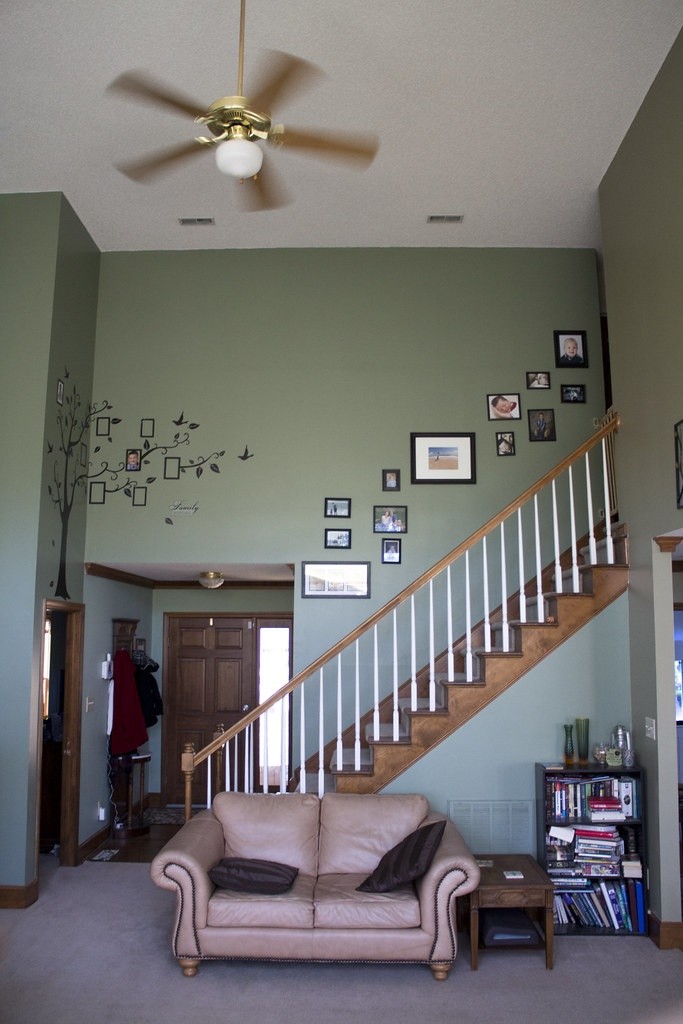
[215, 125, 263, 178]
[199, 571, 224, 588]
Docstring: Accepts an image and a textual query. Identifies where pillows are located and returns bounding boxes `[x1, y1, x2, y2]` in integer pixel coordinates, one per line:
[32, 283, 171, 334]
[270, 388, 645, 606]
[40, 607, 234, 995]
[355, 819, 447, 893]
[207, 857, 299, 894]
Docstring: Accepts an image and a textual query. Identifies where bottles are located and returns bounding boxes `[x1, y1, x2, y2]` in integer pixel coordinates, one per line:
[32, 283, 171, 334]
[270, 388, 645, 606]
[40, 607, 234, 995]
[592, 743, 608, 764]
[564, 725, 575, 765]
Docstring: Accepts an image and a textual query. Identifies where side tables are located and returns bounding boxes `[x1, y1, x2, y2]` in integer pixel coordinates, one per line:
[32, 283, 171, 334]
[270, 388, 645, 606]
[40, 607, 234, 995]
[456, 854, 557, 971]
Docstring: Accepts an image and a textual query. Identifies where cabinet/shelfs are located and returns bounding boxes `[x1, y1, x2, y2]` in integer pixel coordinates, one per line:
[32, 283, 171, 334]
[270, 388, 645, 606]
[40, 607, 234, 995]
[115, 754, 151, 839]
[534, 763, 649, 937]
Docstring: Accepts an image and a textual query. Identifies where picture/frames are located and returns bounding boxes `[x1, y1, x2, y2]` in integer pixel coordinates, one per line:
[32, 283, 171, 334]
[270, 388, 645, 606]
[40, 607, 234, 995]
[487, 393, 521, 421]
[496, 432, 515, 456]
[410, 431, 477, 484]
[553, 330, 589, 369]
[561, 384, 585, 403]
[527, 408, 556, 442]
[301, 468, 408, 600]
[526, 371, 551, 389]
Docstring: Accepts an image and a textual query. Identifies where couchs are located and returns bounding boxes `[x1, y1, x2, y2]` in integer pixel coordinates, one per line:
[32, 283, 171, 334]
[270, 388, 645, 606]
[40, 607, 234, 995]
[150, 792, 481, 981]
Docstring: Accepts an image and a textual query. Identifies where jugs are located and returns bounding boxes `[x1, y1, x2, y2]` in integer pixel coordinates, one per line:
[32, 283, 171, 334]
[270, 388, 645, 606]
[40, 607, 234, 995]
[611, 725, 627, 749]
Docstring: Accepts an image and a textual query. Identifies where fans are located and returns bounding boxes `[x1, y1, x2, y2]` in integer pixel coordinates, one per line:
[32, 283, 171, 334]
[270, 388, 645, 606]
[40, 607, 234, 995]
[106, 0, 380, 213]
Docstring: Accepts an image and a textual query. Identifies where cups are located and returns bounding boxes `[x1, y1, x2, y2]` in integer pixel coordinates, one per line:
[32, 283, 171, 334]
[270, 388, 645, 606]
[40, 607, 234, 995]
[622, 749, 634, 769]
[576, 719, 590, 764]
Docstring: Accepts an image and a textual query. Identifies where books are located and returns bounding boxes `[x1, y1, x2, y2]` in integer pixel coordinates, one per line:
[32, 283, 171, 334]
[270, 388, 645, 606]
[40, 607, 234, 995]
[544, 774, 648, 934]
[476, 860, 495, 868]
[503, 871, 524, 880]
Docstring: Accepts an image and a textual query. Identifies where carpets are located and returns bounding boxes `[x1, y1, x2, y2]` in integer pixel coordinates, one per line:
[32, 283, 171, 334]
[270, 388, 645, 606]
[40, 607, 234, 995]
[143, 808, 206, 824]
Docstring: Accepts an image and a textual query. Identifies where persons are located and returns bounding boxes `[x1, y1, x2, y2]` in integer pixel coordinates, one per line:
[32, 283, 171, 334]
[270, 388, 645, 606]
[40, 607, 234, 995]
[491, 396, 520, 419]
[388, 546, 396, 554]
[560, 338, 583, 364]
[535, 374, 549, 388]
[331, 501, 337, 515]
[380, 509, 404, 532]
[498, 434, 514, 455]
[436, 451, 440, 460]
[536, 412, 546, 437]
[570, 389, 577, 400]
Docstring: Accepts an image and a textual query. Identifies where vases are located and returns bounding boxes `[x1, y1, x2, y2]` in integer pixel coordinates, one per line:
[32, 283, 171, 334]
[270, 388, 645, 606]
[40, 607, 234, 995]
[564, 718, 590, 765]
